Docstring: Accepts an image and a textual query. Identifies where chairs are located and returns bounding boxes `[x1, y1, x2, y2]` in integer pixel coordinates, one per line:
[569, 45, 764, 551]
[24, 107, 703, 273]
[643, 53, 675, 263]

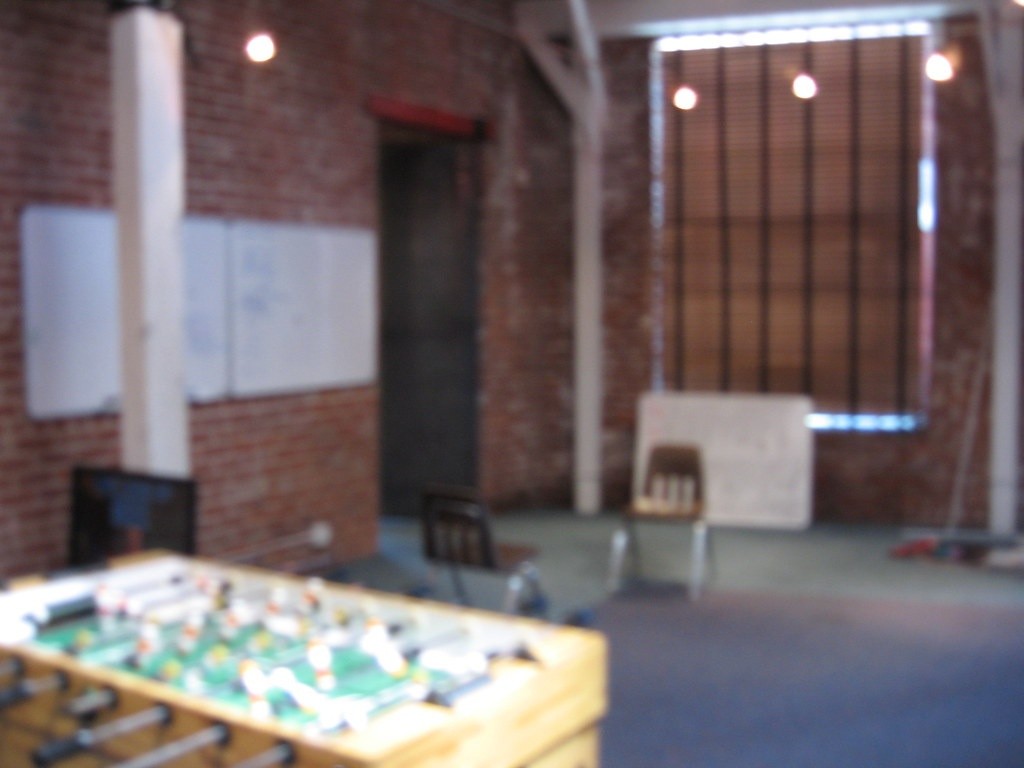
[418, 497, 551, 618]
[610, 443, 718, 600]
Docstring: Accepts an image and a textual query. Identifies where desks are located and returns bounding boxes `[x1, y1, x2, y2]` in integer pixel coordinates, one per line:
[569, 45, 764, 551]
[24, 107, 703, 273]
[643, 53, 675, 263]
[0, 547, 608, 768]
